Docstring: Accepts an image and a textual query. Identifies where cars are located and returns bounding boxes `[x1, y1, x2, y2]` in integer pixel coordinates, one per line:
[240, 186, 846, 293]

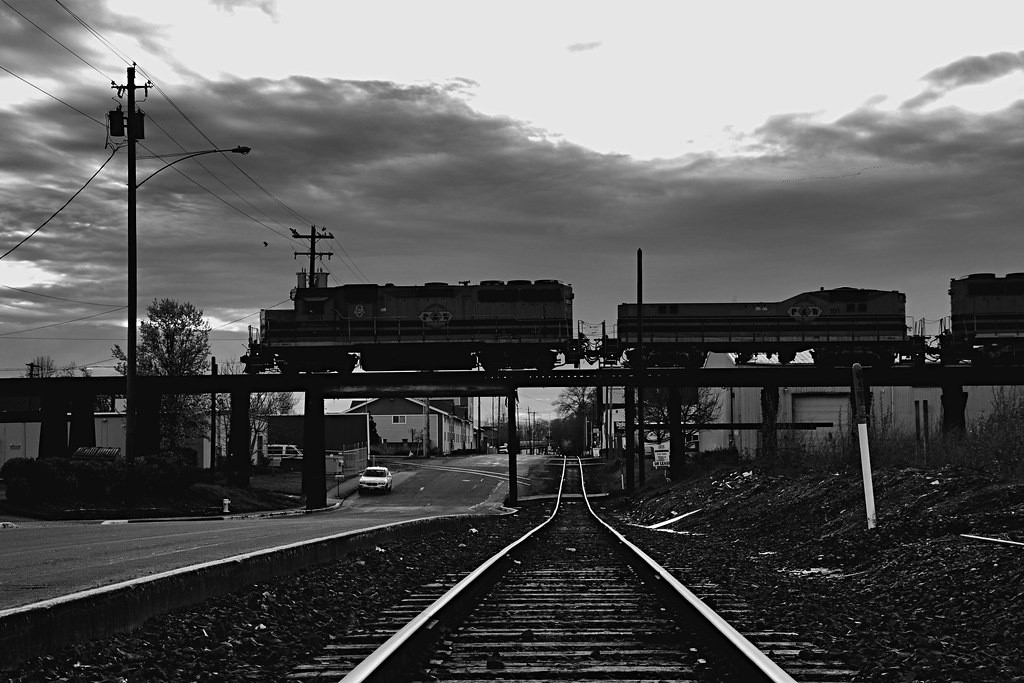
[267, 444, 302, 456]
[499, 446, 508, 454]
[358, 467, 393, 495]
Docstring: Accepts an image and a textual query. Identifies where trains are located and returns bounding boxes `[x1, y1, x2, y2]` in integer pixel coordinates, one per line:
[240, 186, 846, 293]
[240, 273, 1024, 374]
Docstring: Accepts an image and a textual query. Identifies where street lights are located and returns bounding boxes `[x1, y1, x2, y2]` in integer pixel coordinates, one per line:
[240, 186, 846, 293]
[411, 426, 414, 442]
[125, 146, 251, 467]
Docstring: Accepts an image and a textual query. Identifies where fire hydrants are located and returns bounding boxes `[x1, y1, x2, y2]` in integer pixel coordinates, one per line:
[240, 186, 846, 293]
[222, 498, 232, 513]
